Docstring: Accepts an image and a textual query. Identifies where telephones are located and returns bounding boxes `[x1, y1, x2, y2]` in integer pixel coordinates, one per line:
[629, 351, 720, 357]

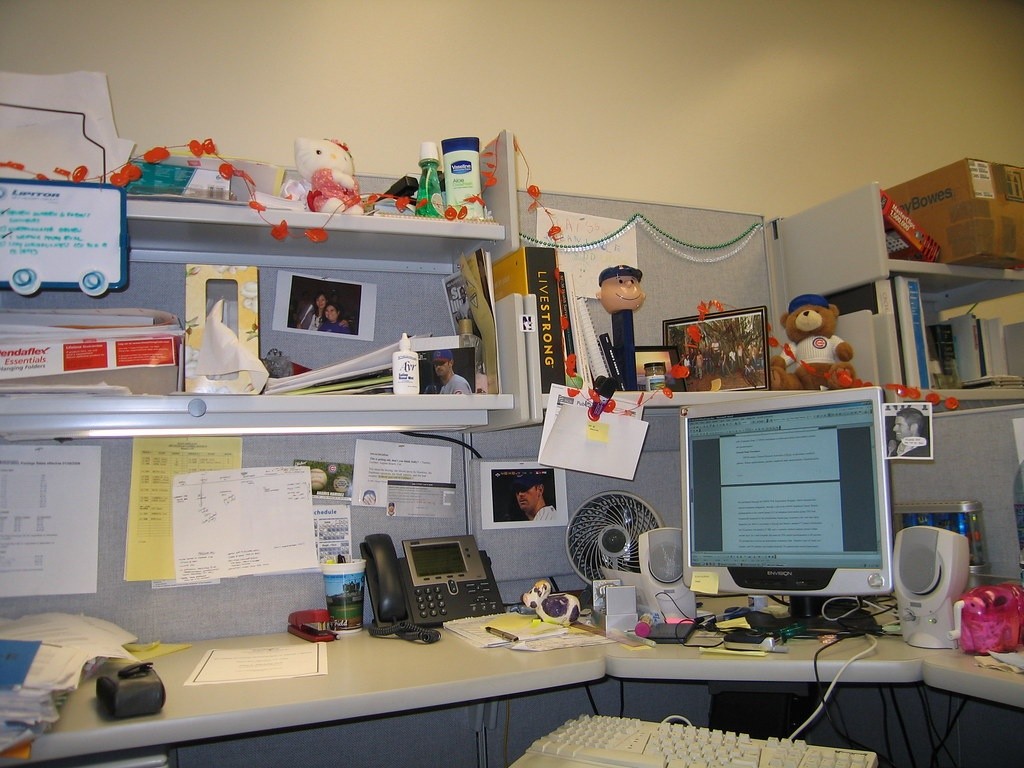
[359, 531, 507, 626]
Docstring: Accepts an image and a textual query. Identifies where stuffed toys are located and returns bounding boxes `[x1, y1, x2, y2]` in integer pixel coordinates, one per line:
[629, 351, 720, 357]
[769, 295, 856, 391]
[294, 137, 363, 215]
[520, 580, 581, 628]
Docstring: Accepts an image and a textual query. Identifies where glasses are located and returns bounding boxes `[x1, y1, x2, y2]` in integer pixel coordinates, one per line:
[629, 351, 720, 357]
[434, 361, 449, 366]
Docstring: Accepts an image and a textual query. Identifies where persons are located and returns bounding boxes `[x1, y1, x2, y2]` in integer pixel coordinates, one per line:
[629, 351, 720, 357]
[514, 475, 556, 521]
[293, 290, 353, 335]
[888, 408, 929, 457]
[388, 503, 395, 516]
[433, 349, 472, 394]
[682, 336, 765, 378]
[595, 264, 646, 315]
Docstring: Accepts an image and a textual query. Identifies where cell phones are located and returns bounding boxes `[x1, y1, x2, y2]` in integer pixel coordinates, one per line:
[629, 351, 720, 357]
[724, 632, 789, 653]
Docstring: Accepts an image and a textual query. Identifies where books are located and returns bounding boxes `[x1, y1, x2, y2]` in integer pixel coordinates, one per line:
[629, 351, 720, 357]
[559, 269, 627, 393]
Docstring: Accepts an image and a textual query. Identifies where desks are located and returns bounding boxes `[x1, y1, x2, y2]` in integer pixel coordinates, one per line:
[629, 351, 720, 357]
[0, 605, 1024, 768]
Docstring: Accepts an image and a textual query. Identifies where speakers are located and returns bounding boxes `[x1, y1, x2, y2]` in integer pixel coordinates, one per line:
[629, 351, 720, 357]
[638, 527, 698, 622]
[892, 525, 970, 649]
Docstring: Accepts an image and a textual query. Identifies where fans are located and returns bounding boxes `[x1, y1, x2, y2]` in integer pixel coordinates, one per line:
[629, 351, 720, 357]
[564, 490, 665, 609]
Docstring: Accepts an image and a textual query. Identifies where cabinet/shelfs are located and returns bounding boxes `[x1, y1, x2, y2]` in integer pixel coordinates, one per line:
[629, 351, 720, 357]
[0, 124, 891, 437]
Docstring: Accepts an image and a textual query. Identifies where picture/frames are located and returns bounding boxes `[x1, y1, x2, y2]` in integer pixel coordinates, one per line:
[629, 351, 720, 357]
[662, 305, 770, 393]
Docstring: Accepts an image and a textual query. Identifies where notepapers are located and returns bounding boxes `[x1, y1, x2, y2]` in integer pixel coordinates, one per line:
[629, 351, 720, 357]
[715, 616, 751, 632]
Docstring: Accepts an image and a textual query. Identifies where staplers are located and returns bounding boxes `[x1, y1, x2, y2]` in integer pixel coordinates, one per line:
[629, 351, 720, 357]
[287, 608, 336, 643]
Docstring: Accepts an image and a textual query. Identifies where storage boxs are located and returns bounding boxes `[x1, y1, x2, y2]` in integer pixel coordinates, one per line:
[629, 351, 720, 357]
[879, 188, 941, 261]
[0, 335, 183, 394]
[885, 158, 1024, 268]
[891, 276, 931, 389]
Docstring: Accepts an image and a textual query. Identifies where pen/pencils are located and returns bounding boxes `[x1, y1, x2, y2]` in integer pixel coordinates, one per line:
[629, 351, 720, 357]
[485, 627, 519, 641]
[330, 627, 342, 642]
[610, 627, 656, 647]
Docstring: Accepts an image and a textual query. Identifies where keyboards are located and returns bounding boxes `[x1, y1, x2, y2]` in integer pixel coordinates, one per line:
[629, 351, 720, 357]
[508, 715, 878, 768]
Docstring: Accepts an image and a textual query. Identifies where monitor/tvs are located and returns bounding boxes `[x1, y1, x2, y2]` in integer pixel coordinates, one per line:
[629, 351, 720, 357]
[680, 385, 894, 639]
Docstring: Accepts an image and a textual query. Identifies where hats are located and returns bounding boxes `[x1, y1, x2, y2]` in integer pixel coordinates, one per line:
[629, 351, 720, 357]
[511, 475, 542, 493]
[432, 349, 452, 359]
